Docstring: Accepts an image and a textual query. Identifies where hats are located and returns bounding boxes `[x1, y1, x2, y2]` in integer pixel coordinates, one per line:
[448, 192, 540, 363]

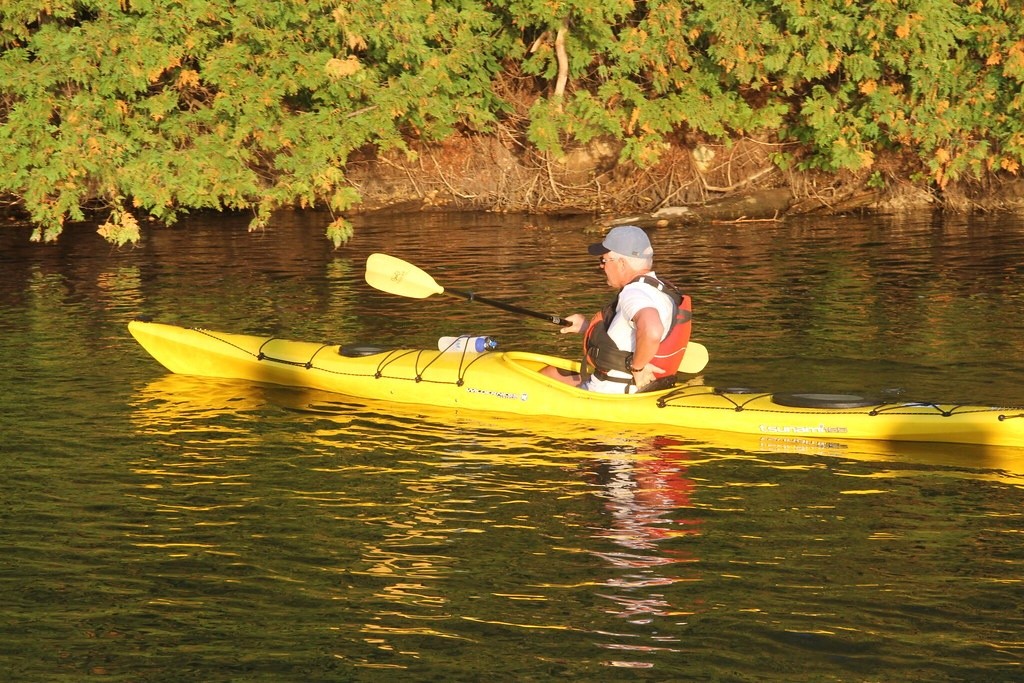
[588, 225, 654, 258]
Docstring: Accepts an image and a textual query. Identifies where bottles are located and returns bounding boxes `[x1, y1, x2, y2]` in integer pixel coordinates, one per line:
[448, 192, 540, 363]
[438, 334, 497, 352]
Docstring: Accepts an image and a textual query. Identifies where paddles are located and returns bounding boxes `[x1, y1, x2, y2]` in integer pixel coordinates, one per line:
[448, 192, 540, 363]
[364, 252, 708, 374]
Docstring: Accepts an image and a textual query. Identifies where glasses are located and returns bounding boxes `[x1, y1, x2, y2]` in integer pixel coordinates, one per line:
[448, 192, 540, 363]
[600, 257, 619, 264]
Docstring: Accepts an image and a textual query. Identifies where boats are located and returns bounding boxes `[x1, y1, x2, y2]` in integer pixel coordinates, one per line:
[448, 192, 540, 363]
[125, 318, 1024, 449]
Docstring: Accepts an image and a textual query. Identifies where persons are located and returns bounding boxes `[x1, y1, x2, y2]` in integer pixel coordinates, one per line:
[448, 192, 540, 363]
[560, 226, 691, 398]
